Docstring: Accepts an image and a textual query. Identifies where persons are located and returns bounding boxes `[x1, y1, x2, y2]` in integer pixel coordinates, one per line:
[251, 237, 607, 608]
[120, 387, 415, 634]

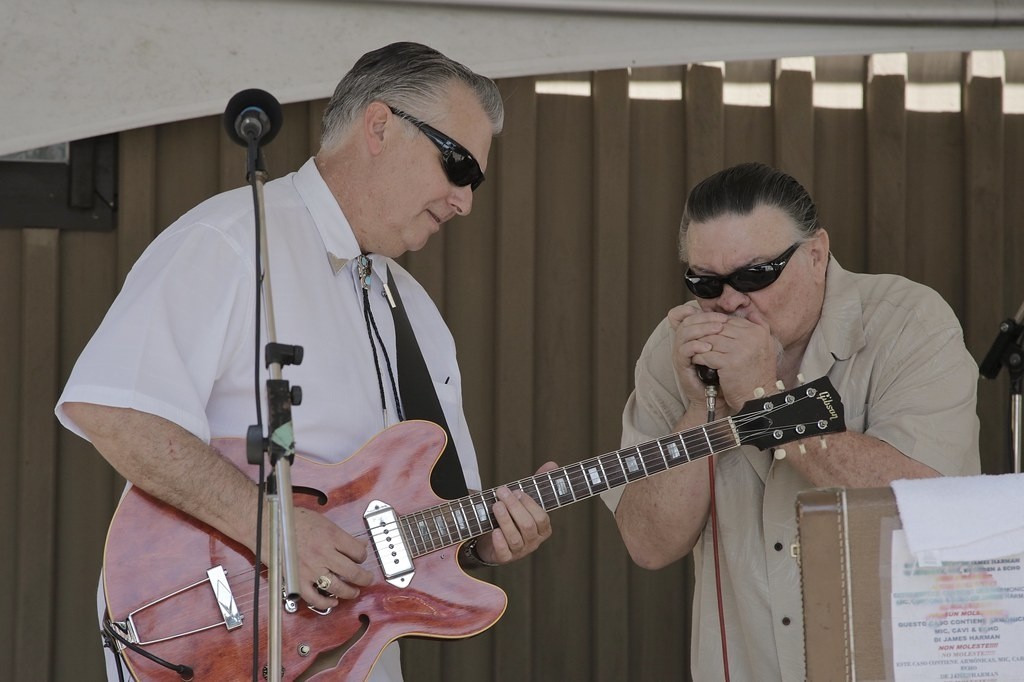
[601, 162, 981, 682]
[54, 40, 559, 682]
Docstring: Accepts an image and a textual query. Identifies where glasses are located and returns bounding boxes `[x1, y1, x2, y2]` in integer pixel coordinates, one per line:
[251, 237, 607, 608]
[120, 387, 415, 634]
[387, 106, 486, 192]
[684, 240, 803, 299]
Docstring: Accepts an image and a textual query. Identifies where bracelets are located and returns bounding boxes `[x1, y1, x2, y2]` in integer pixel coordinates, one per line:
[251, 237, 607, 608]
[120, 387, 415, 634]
[463, 537, 500, 568]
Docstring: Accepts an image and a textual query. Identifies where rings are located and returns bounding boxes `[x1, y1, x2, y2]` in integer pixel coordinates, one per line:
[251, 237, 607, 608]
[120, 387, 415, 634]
[316, 570, 335, 591]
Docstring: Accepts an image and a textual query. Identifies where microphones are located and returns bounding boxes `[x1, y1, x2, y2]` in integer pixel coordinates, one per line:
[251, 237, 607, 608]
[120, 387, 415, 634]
[695, 363, 720, 412]
[223, 88, 284, 148]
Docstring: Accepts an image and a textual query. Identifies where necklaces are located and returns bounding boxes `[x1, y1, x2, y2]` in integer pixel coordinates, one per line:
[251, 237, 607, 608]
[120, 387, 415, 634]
[355, 256, 402, 432]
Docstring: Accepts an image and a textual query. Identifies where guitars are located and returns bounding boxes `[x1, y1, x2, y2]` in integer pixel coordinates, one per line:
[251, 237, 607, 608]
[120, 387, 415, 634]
[100, 374, 848, 682]
